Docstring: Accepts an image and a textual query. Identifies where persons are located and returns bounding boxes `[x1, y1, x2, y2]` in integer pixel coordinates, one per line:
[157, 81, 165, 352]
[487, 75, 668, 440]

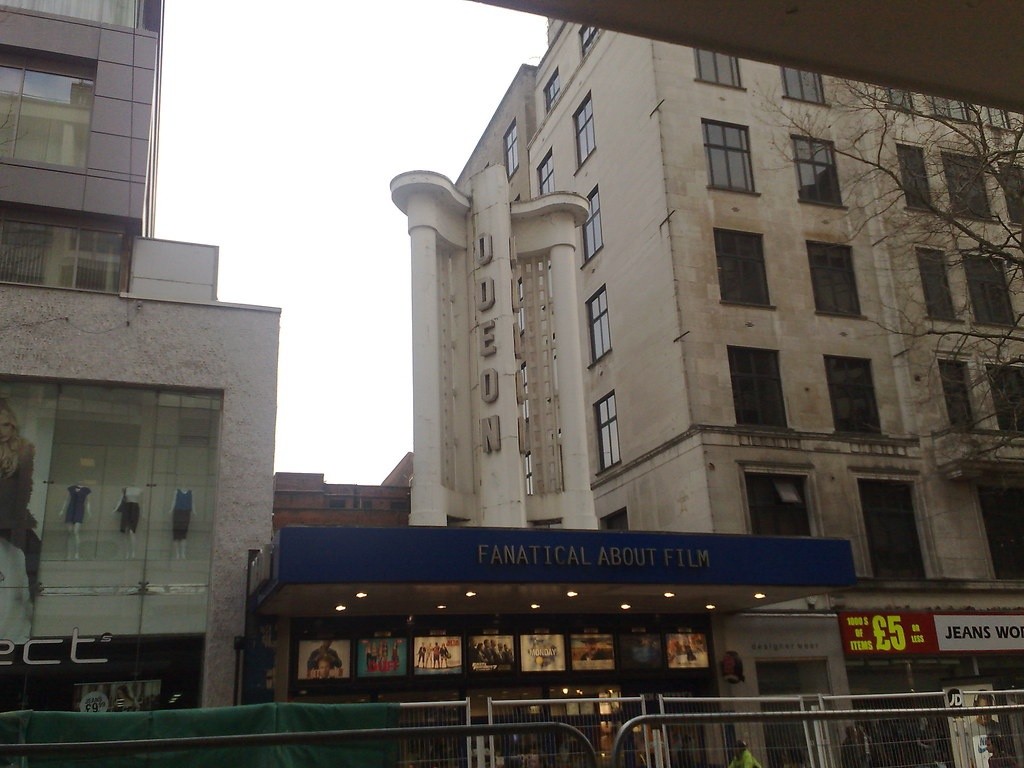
[580, 638, 605, 660]
[57, 483, 91, 560]
[727, 740, 762, 768]
[167, 488, 198, 559]
[915, 717, 938, 765]
[112, 486, 146, 559]
[307, 640, 341, 678]
[470, 638, 514, 664]
[842, 727, 864, 768]
[969, 690, 1002, 734]
[416, 642, 450, 668]
[668, 638, 698, 663]
[0, 408, 41, 591]
[980, 734, 1017, 768]
[366, 640, 397, 671]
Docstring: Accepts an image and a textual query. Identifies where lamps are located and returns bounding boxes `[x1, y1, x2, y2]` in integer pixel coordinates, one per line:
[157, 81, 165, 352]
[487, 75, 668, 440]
[775, 476, 802, 504]
